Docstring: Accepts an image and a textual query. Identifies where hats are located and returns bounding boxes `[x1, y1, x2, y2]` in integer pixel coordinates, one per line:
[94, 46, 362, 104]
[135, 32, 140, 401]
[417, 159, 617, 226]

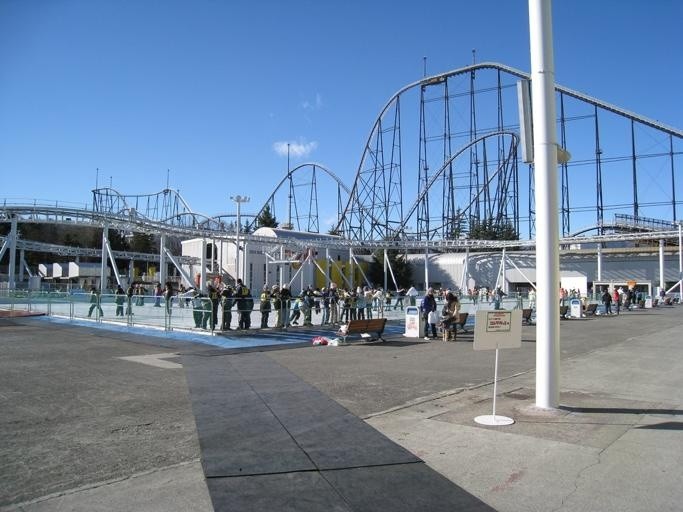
[356, 286, 369, 293]
[263, 283, 289, 290]
[427, 287, 433, 292]
[330, 282, 337, 289]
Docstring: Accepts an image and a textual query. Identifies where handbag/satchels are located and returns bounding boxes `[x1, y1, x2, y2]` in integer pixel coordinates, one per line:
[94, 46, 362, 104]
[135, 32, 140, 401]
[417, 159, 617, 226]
[427, 310, 440, 324]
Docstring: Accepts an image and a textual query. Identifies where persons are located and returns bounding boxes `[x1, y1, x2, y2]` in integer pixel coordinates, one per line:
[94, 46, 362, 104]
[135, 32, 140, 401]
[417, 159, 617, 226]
[468, 285, 506, 309]
[616, 287, 643, 305]
[289, 282, 385, 326]
[186, 278, 254, 330]
[260, 283, 292, 328]
[559, 287, 582, 307]
[440, 293, 460, 339]
[384, 285, 419, 311]
[527, 285, 536, 309]
[516, 286, 524, 310]
[112, 280, 187, 317]
[438, 288, 452, 300]
[600, 289, 612, 314]
[613, 288, 620, 315]
[85, 288, 103, 317]
[421, 287, 439, 340]
[659, 288, 665, 302]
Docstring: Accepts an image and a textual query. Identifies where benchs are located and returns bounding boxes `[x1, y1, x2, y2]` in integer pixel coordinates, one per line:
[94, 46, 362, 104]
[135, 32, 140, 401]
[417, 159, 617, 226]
[560, 306, 568, 320]
[440, 313, 468, 332]
[639, 297, 679, 309]
[522, 309, 532, 323]
[336, 318, 387, 343]
[587, 304, 598, 315]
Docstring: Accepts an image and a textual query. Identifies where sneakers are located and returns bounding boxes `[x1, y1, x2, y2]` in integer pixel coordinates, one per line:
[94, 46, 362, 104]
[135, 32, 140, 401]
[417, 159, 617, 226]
[260, 323, 269, 328]
[292, 321, 313, 326]
[423, 335, 456, 341]
[219, 325, 249, 330]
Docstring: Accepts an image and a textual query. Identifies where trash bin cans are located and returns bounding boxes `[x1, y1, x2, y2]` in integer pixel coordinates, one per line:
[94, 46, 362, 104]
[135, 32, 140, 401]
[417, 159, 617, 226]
[404, 306, 424, 338]
[570, 299, 582, 318]
[644, 295, 652, 309]
